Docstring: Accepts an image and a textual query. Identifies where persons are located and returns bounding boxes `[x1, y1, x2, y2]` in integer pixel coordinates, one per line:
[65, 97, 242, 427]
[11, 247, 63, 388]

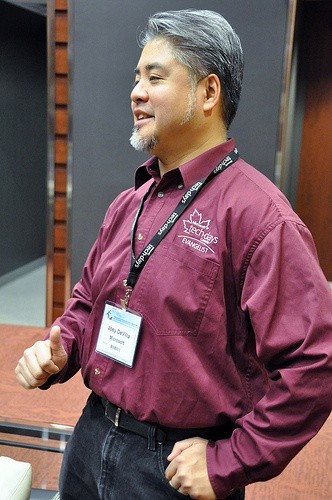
[9, 7, 332, 500]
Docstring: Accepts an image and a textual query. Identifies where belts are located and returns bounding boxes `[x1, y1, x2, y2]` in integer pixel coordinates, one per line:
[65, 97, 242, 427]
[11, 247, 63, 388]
[100, 398, 225, 444]
